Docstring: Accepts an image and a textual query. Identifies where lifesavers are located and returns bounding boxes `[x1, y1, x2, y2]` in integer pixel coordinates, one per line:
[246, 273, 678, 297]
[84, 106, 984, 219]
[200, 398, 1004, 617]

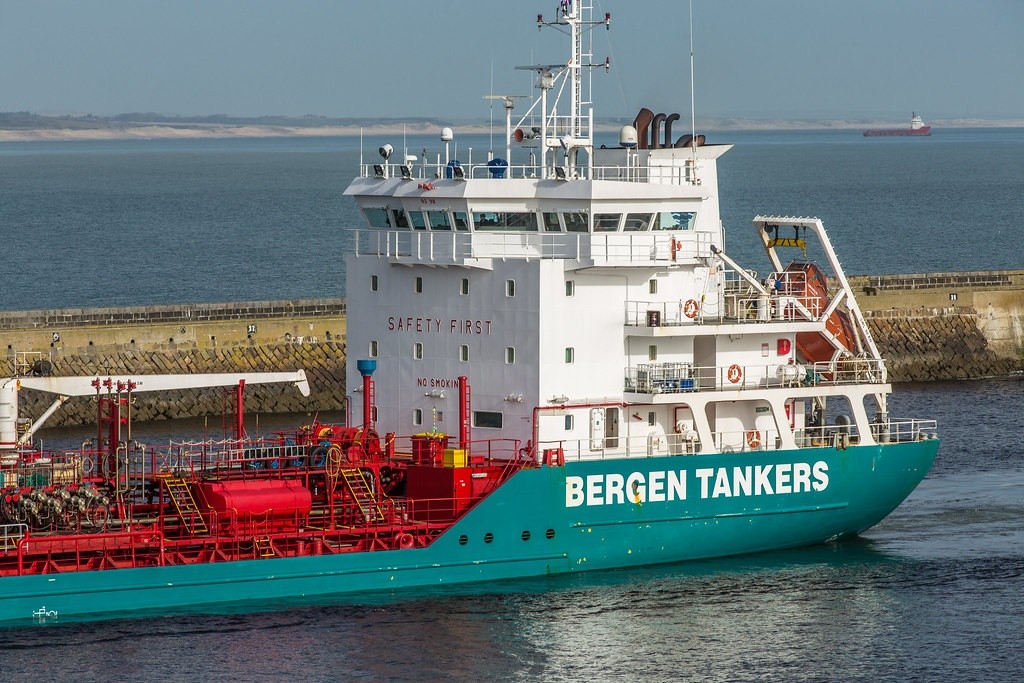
[747, 428, 761, 447]
[311, 446, 328, 466]
[728, 366, 741, 382]
[244, 449, 264, 470]
[286, 444, 307, 468]
[265, 446, 287, 469]
[685, 299, 698, 317]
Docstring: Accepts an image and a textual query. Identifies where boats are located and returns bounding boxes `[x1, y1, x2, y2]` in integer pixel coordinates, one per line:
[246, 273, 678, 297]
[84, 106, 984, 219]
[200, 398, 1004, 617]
[862, 110, 933, 136]
[1, 0, 942, 628]
